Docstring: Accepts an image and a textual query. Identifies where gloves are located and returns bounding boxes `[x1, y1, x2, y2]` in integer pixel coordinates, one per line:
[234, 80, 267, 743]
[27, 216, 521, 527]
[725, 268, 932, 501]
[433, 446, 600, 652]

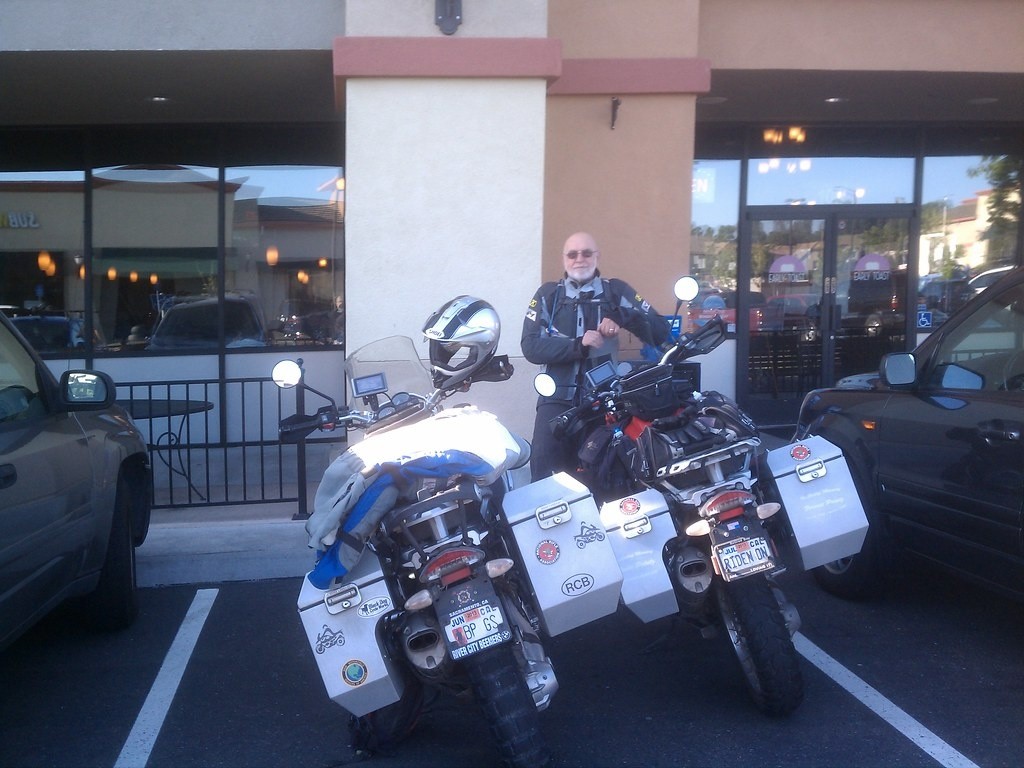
[653, 406, 710, 445]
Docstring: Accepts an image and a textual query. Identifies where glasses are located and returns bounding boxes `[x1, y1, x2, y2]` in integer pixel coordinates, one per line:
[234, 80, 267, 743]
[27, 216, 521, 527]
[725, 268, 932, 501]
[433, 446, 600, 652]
[563, 249, 598, 259]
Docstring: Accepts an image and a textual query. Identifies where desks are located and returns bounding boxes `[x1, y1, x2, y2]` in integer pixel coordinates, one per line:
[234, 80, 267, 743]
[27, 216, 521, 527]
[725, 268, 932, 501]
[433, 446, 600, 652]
[110, 398, 212, 500]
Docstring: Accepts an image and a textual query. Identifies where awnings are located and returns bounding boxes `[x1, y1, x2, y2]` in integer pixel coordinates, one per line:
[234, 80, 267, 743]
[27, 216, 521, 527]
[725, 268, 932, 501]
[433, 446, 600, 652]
[92, 250, 218, 279]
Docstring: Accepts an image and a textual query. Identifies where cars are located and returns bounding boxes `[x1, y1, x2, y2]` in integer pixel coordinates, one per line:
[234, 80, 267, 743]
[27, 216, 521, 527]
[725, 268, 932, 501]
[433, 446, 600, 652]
[1, 310, 156, 658]
[684, 264, 1017, 344]
[790, 269, 1024, 622]
[0, 290, 340, 353]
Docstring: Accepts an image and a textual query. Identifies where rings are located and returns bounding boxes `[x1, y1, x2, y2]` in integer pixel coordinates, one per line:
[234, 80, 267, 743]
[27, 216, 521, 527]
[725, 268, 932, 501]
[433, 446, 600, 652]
[609, 328, 614, 331]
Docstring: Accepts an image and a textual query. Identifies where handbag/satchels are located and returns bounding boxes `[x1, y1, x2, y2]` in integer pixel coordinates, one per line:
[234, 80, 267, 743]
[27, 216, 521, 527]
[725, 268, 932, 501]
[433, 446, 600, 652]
[636, 389, 759, 486]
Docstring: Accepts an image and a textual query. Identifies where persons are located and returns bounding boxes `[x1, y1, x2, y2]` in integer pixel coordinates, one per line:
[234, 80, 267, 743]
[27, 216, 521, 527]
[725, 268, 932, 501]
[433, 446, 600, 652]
[520, 232, 671, 482]
[128, 327, 141, 341]
[320, 295, 344, 345]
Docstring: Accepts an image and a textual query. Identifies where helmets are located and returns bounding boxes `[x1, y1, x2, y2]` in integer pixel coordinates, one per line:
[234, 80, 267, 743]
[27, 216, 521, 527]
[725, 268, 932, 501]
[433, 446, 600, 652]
[421, 294, 501, 390]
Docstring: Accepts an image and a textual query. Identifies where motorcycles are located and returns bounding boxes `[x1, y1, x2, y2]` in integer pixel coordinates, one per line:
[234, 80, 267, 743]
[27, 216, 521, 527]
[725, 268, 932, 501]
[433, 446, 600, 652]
[532, 275, 870, 720]
[270, 335, 623, 768]
